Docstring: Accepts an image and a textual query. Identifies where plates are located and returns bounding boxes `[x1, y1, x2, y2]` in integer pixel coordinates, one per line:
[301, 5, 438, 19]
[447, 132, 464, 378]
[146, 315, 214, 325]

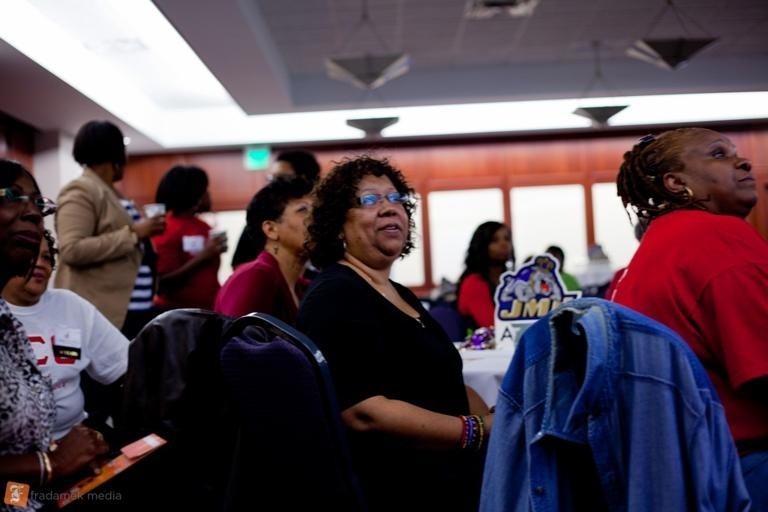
[458, 347, 496, 361]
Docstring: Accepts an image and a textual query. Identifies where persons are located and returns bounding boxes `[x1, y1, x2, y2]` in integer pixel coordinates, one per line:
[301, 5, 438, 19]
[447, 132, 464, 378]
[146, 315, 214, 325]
[292, 155, 502, 507]
[544, 245, 580, 291]
[457, 220, 514, 330]
[0, 146, 326, 511]
[609, 125, 767, 460]
[52, 120, 168, 340]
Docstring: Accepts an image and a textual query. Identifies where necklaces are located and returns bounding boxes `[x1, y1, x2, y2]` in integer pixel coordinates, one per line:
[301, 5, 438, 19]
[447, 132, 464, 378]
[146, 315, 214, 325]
[342, 258, 426, 329]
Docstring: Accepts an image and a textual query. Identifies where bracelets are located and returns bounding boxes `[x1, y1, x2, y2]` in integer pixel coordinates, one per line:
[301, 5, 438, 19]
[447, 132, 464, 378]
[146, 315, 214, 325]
[458, 414, 485, 450]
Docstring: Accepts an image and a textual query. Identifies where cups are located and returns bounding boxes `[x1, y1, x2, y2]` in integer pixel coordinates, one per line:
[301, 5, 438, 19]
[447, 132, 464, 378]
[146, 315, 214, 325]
[143, 202, 166, 233]
[208, 226, 228, 253]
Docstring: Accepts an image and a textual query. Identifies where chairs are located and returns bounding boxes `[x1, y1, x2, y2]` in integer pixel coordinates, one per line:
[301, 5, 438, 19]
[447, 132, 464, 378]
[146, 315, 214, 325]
[119, 306, 235, 511]
[219, 313, 360, 511]
[479, 296, 751, 511]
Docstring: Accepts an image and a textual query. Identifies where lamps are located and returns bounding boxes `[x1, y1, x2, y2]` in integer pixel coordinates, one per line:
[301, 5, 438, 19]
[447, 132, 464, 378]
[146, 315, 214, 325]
[345, 89, 398, 139]
[573, 42, 627, 130]
[325, 0, 407, 88]
[626, 2, 720, 72]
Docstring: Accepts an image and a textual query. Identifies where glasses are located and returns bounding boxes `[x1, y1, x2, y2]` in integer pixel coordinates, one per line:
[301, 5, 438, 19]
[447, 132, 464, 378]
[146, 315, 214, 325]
[0, 189, 56, 215]
[353, 193, 405, 205]
